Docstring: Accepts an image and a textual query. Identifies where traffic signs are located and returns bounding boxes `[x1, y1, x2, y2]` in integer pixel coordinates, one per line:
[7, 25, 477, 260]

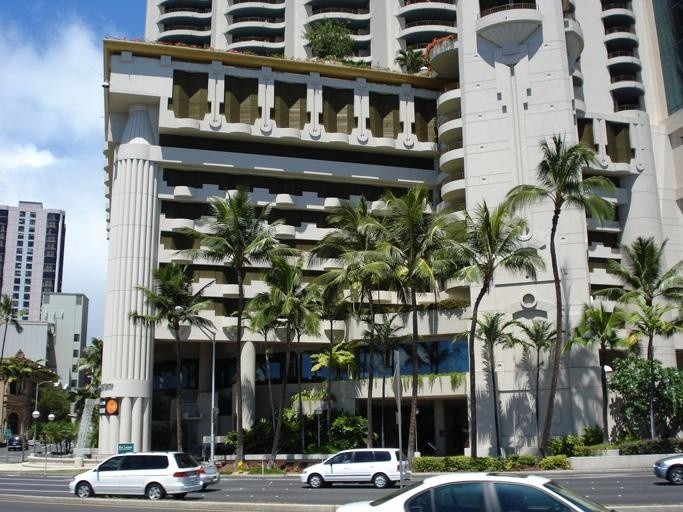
[117, 443, 133, 455]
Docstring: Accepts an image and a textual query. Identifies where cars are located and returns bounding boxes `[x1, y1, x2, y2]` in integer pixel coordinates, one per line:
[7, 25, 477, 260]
[652, 454, 682, 486]
[333, 469, 622, 512]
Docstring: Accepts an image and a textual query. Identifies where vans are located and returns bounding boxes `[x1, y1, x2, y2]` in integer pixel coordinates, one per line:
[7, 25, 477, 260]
[7, 434, 28, 452]
[299, 447, 411, 489]
[67, 449, 204, 501]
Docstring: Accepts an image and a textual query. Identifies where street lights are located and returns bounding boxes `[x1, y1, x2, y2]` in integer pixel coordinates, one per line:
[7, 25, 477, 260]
[31, 380, 54, 453]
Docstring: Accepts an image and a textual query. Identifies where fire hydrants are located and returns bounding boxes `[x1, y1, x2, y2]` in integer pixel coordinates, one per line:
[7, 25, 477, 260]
[238, 459, 246, 475]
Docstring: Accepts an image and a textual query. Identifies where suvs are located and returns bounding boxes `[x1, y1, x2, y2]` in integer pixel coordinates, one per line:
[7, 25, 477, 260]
[192, 455, 220, 493]
